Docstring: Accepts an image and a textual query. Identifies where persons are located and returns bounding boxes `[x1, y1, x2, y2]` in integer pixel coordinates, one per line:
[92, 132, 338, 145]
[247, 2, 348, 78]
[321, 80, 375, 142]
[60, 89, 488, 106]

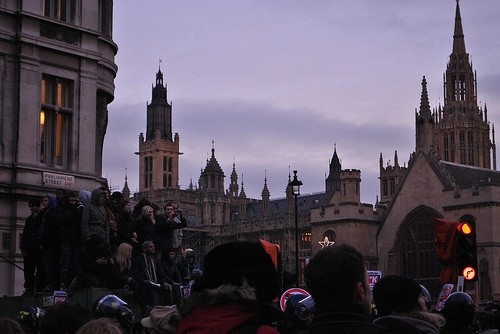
[300, 244, 400, 333]
[0, 186, 500, 334]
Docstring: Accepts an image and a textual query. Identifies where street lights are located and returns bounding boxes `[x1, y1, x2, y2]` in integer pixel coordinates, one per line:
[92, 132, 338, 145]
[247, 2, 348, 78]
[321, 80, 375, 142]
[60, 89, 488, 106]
[289, 170, 303, 289]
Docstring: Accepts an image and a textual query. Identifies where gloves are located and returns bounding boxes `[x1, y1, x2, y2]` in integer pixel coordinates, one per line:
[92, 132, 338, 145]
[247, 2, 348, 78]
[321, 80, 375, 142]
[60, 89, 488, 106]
[87, 239, 93, 245]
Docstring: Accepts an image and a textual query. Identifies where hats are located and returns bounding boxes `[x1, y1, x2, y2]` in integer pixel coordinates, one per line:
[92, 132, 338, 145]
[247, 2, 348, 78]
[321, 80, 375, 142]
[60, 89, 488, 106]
[184, 248, 195, 260]
[28, 199, 40, 207]
[373, 275, 418, 313]
[0, 317, 25, 334]
[141, 240, 152, 251]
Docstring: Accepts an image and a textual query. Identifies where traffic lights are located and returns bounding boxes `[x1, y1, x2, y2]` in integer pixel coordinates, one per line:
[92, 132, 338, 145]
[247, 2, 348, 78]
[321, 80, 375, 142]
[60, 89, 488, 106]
[433, 217, 457, 284]
[457, 213, 479, 282]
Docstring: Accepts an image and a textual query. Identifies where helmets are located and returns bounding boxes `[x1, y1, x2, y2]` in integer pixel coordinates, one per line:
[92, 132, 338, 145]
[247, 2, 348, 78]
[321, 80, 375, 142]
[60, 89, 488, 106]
[444, 292, 476, 326]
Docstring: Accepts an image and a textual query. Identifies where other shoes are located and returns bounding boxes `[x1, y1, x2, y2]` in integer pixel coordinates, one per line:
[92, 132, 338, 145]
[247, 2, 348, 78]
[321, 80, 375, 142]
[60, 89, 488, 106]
[21, 288, 33, 297]
